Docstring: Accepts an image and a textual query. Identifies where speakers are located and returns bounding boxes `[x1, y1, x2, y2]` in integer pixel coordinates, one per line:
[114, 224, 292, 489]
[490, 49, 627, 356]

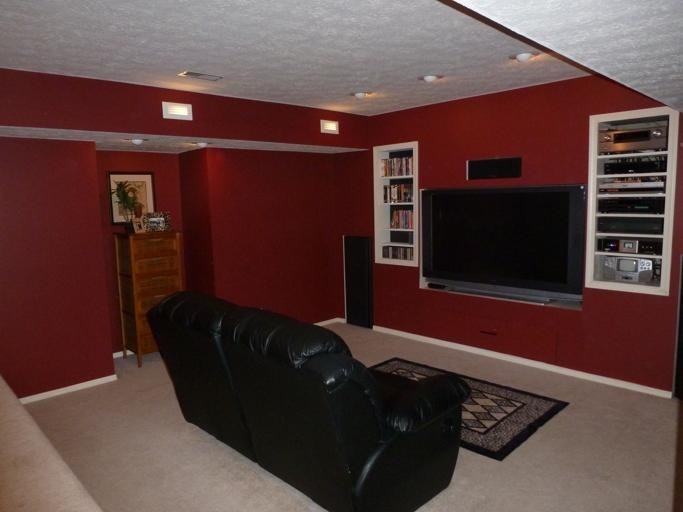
[342, 234, 373, 328]
[466, 156, 521, 181]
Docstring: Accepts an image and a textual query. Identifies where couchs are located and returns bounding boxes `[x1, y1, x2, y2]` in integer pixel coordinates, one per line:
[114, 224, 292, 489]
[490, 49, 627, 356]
[145, 290, 472, 512]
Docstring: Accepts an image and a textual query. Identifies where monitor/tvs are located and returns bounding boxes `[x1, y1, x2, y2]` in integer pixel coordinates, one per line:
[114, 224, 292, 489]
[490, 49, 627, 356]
[418, 184, 588, 304]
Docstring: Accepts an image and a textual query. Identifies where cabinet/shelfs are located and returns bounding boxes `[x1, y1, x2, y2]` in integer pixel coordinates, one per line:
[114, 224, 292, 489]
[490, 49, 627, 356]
[584, 105, 679, 297]
[112, 230, 186, 368]
[373, 140, 420, 268]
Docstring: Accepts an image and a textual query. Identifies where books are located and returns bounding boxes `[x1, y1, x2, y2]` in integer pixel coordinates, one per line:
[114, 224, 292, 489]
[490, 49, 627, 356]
[382, 246, 413, 260]
[380, 157, 414, 229]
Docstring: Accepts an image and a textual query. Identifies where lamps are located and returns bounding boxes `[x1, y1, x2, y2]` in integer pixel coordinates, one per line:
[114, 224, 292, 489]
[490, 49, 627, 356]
[516, 52, 532, 63]
[353, 92, 366, 99]
[423, 75, 438, 84]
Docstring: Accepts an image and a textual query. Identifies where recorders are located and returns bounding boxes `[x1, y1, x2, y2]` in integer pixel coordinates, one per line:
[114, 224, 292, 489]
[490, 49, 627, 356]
[594, 256, 653, 283]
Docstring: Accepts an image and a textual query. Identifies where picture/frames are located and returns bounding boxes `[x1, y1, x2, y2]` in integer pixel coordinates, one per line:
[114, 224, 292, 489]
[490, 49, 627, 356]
[108, 171, 156, 225]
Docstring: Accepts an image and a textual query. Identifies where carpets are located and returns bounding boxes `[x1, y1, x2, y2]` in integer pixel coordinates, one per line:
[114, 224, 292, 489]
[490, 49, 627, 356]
[367, 357, 570, 461]
